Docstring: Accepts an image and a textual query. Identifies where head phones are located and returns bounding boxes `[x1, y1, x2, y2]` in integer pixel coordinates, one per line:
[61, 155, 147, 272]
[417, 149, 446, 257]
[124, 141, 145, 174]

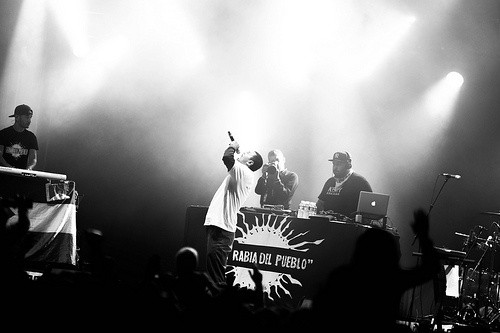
[346, 151, 352, 169]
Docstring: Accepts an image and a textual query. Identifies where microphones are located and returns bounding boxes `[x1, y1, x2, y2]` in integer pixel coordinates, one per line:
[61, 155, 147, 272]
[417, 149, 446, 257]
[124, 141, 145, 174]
[441, 173, 462, 180]
[228, 131, 240, 154]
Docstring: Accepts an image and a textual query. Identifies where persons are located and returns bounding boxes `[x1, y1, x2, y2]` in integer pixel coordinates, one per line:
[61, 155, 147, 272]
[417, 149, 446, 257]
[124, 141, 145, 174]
[316, 152, 372, 219]
[0, 209, 441, 333]
[255, 150, 299, 213]
[205, 142, 263, 290]
[0, 104, 39, 231]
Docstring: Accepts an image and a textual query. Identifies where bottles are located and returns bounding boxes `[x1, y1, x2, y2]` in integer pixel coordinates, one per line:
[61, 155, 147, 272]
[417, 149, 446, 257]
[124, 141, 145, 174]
[296, 200, 317, 219]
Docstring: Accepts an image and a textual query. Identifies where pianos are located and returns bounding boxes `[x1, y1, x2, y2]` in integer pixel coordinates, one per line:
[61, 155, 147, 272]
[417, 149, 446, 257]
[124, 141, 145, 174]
[0, 166, 68, 204]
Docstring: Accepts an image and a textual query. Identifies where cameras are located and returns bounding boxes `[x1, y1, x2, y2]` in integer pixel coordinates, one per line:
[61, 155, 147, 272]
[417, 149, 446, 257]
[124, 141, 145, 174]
[266, 163, 278, 174]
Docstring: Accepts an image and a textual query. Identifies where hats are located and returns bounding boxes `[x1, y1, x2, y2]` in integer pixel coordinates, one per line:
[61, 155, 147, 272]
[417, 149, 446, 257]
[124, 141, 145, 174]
[8, 104, 33, 117]
[328, 151, 351, 162]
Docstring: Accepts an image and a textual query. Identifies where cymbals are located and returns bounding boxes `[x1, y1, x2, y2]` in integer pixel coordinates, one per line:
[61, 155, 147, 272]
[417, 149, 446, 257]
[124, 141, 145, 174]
[479, 212, 500, 216]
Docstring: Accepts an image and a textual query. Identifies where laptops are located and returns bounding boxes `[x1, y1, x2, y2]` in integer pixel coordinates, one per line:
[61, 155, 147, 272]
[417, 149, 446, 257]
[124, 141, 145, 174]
[351, 191, 389, 220]
